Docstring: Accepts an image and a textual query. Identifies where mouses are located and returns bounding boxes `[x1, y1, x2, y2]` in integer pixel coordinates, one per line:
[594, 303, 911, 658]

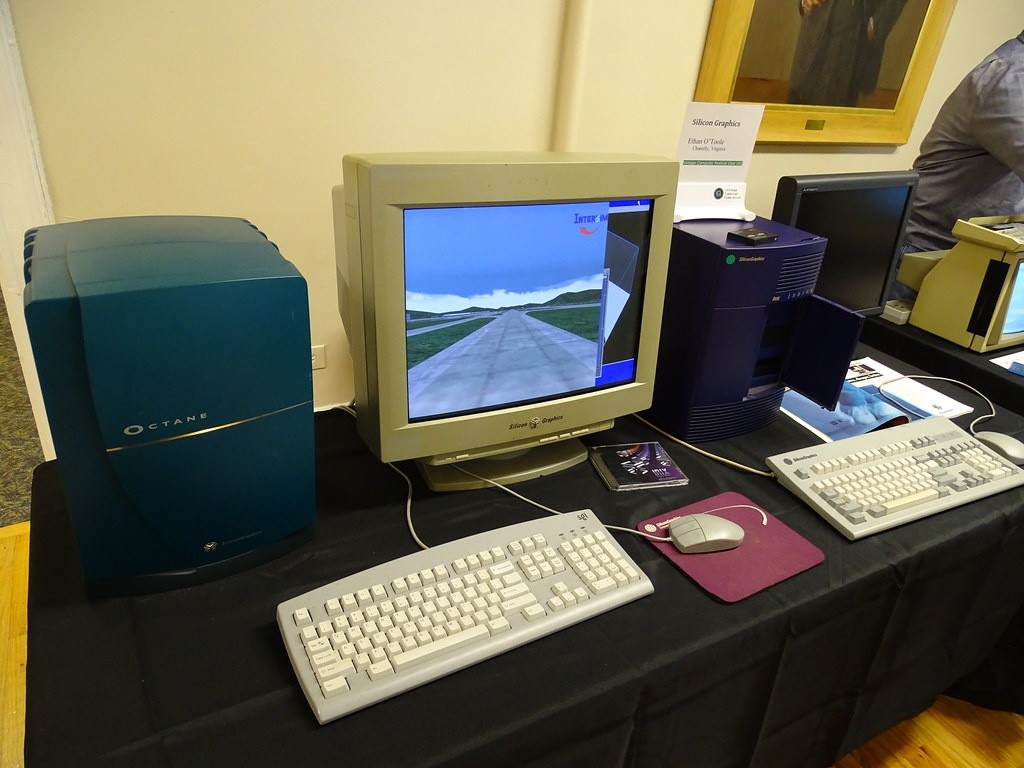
[669, 513, 745, 554]
[974, 431, 1024, 465]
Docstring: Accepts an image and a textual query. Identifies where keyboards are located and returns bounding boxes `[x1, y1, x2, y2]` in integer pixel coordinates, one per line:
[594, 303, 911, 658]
[765, 414, 1024, 541]
[275, 508, 656, 724]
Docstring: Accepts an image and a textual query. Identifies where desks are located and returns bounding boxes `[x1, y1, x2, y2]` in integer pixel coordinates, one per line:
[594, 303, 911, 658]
[859, 314, 1024, 417]
[25, 339, 1024, 768]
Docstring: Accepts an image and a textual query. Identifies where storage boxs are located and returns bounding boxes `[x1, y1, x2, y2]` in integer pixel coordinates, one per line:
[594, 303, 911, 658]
[634, 213, 866, 444]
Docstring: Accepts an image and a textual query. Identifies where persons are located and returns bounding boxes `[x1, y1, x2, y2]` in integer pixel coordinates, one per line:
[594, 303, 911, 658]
[888, 31, 1024, 301]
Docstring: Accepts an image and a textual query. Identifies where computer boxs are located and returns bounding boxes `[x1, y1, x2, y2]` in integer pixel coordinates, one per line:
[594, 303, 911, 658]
[23, 218, 318, 599]
[636, 212, 866, 445]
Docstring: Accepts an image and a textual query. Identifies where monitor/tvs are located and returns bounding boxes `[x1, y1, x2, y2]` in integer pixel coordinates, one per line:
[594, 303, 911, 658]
[897, 214, 1024, 352]
[771, 171, 920, 316]
[332, 155, 678, 493]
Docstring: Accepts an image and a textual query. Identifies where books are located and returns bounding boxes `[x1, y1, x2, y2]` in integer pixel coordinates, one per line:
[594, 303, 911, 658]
[779, 356, 973, 442]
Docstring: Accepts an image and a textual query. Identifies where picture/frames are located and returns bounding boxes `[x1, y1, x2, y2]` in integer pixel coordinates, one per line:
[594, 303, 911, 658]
[693, 0, 958, 144]
